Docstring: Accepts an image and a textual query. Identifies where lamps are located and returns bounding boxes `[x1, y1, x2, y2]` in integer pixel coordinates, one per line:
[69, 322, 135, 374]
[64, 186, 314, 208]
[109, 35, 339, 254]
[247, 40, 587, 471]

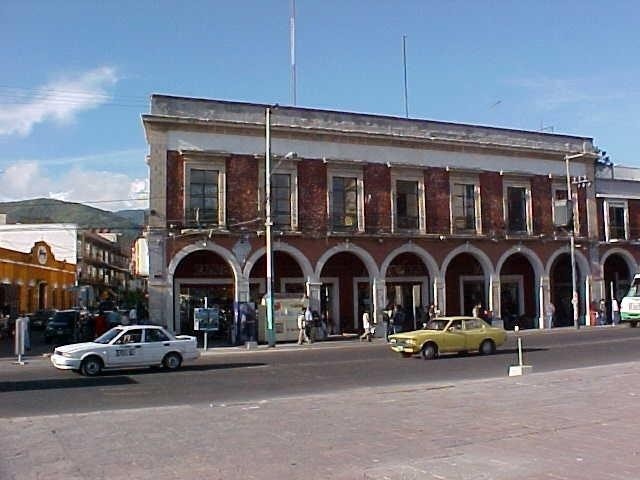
[384, 160, 392, 169]
[445, 165, 451, 173]
[323, 157, 329, 164]
[500, 167, 505, 176]
[202, 235, 208, 248]
[170, 222, 183, 230]
[548, 172, 552, 180]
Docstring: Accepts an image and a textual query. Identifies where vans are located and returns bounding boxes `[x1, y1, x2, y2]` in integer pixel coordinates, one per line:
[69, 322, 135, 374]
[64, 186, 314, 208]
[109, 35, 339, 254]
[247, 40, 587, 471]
[618, 271, 640, 330]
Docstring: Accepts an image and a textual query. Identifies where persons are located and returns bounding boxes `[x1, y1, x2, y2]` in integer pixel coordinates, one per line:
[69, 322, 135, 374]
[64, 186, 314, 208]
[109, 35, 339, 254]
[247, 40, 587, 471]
[472, 303, 480, 318]
[20, 311, 33, 350]
[421, 305, 431, 328]
[429, 303, 437, 322]
[130, 305, 137, 323]
[479, 303, 490, 323]
[296, 304, 312, 346]
[391, 304, 406, 333]
[120, 310, 130, 325]
[108, 308, 119, 328]
[610, 297, 620, 327]
[360, 309, 377, 343]
[96, 311, 107, 337]
[598, 298, 608, 325]
[304, 307, 312, 339]
[381, 302, 394, 343]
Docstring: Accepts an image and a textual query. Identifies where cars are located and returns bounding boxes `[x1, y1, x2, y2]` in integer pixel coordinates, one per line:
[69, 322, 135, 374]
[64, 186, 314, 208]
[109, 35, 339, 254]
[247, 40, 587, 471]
[387, 316, 509, 358]
[49, 323, 202, 377]
[25, 306, 132, 344]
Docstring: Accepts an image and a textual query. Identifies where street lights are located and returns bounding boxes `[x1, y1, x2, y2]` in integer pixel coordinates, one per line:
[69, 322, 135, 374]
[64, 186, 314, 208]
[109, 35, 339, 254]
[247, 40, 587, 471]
[262, 150, 303, 348]
[565, 151, 603, 329]
